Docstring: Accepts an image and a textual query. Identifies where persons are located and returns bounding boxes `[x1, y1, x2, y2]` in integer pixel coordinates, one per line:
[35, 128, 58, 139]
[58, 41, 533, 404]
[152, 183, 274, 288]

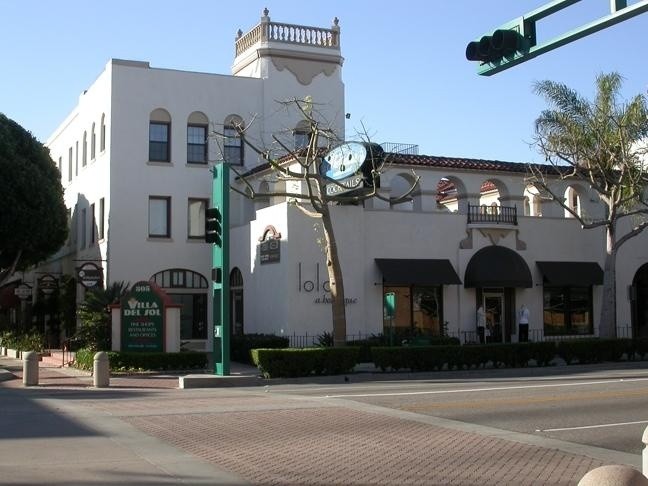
[477, 305, 485, 342]
[518, 304, 530, 342]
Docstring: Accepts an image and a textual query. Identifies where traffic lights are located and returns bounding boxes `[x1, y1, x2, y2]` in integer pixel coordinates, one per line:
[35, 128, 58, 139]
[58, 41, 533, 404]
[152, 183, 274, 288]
[465, 28, 521, 62]
[200, 206, 220, 245]
